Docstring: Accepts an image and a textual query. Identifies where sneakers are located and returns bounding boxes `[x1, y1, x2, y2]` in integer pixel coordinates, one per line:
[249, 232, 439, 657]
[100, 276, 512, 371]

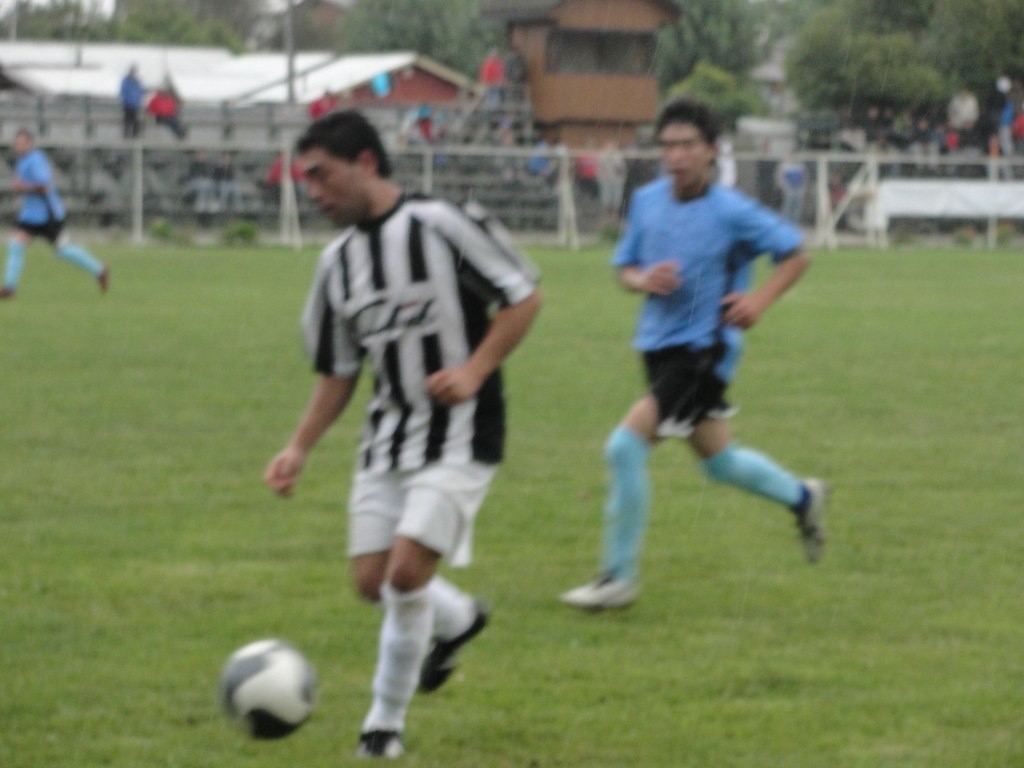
[796, 477, 826, 562]
[559, 576, 636, 606]
[421, 611, 488, 694]
[99, 265, 110, 290]
[356, 731, 405, 758]
[0, 287, 13, 297]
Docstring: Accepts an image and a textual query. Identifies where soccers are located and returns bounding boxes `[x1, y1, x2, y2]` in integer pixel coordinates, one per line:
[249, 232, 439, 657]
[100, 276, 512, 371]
[222, 638, 315, 738]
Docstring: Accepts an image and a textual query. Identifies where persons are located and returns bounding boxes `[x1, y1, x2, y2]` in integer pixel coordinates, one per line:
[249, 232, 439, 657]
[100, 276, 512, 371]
[556, 95, 827, 619]
[118, 62, 143, 139]
[1, 131, 113, 299]
[151, 69, 186, 140]
[268, 112, 545, 765]
[835, 64, 1024, 179]
[473, 41, 621, 206]
[770, 146, 807, 222]
